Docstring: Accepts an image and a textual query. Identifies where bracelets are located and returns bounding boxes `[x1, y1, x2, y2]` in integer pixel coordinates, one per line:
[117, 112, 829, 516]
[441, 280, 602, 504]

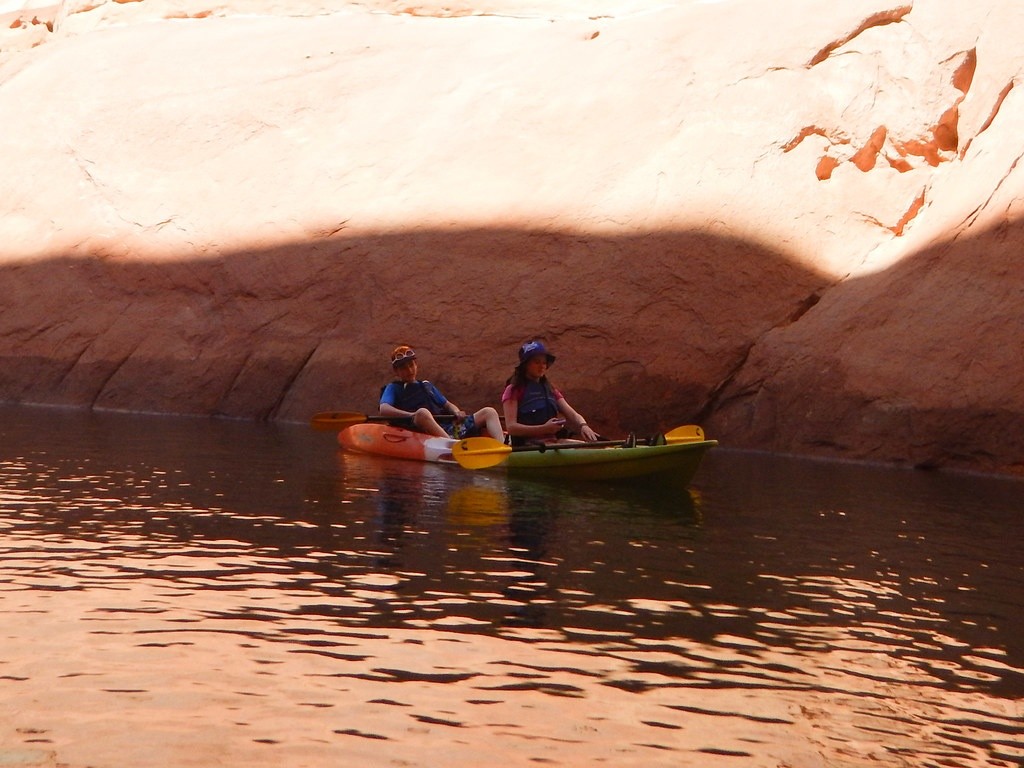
[580, 423, 589, 427]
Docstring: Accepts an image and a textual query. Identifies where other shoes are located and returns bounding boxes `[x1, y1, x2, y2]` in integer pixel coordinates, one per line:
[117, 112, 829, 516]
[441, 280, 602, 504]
[622, 431, 636, 448]
[651, 432, 666, 446]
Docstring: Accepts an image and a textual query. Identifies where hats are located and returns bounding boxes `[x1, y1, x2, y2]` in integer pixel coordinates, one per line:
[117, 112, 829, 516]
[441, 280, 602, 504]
[514, 342, 556, 370]
[391, 346, 418, 366]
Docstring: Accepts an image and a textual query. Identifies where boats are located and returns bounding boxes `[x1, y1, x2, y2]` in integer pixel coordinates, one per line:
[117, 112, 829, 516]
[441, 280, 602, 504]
[338, 422, 719, 476]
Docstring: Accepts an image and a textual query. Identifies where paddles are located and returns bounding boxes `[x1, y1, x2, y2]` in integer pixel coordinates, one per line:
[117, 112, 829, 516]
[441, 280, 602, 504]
[450, 424, 705, 470]
[308, 410, 504, 431]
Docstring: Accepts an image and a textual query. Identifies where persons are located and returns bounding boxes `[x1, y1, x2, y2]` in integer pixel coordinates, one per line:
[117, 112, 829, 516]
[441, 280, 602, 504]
[501, 339, 668, 450]
[376, 347, 516, 449]
